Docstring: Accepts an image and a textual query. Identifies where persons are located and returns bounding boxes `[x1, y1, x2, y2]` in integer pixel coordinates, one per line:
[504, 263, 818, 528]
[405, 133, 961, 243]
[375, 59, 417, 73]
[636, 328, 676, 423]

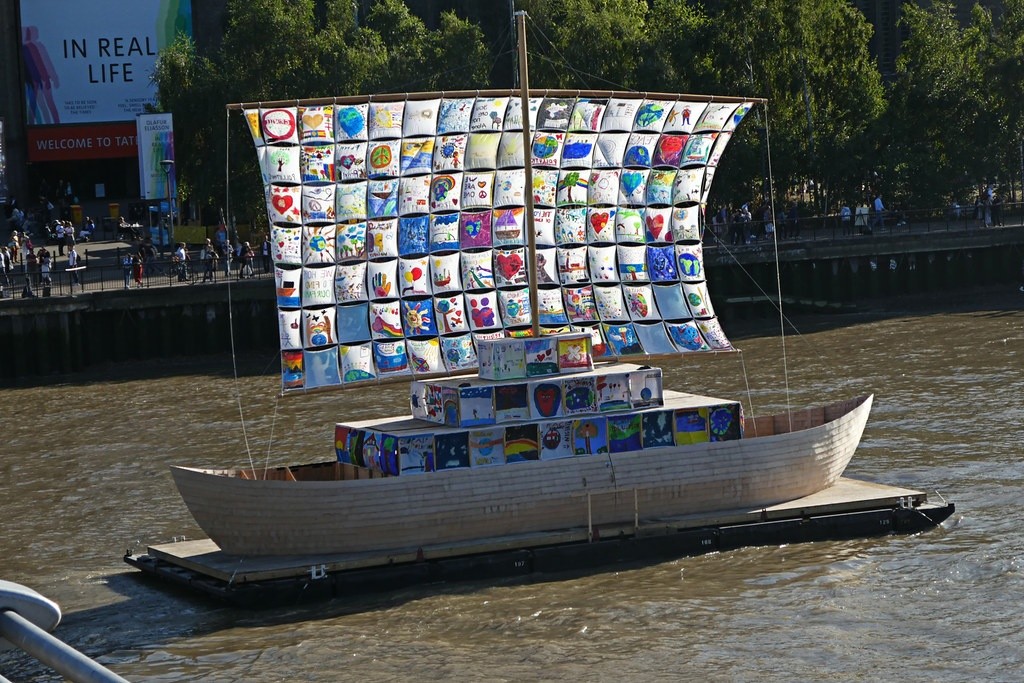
[0, 217, 272, 298]
[700, 185, 1003, 245]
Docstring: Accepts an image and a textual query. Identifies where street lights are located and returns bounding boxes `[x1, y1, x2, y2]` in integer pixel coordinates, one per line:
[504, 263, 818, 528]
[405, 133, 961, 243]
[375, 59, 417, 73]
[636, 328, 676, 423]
[158, 159, 175, 257]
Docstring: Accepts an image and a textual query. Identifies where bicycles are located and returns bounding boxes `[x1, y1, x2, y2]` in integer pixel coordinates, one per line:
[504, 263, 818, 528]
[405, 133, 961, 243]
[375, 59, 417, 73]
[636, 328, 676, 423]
[162, 260, 199, 285]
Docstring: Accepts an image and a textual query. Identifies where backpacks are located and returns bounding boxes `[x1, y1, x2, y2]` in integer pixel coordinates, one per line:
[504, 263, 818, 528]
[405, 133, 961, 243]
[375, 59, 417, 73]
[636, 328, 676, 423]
[71, 251, 82, 265]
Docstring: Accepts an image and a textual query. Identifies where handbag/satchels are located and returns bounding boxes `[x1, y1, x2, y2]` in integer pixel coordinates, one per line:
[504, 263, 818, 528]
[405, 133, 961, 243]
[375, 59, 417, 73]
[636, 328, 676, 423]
[8, 259, 14, 271]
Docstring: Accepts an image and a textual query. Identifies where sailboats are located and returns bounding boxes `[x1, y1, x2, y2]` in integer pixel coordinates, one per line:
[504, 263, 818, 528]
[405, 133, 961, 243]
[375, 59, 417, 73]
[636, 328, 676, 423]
[124, 0, 956, 610]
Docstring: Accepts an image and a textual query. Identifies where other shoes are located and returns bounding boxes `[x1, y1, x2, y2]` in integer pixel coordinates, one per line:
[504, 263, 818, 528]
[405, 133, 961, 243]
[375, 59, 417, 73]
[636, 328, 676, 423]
[141, 281, 144, 287]
[178, 279, 185, 282]
[183, 278, 190, 281]
[137, 285, 140, 288]
